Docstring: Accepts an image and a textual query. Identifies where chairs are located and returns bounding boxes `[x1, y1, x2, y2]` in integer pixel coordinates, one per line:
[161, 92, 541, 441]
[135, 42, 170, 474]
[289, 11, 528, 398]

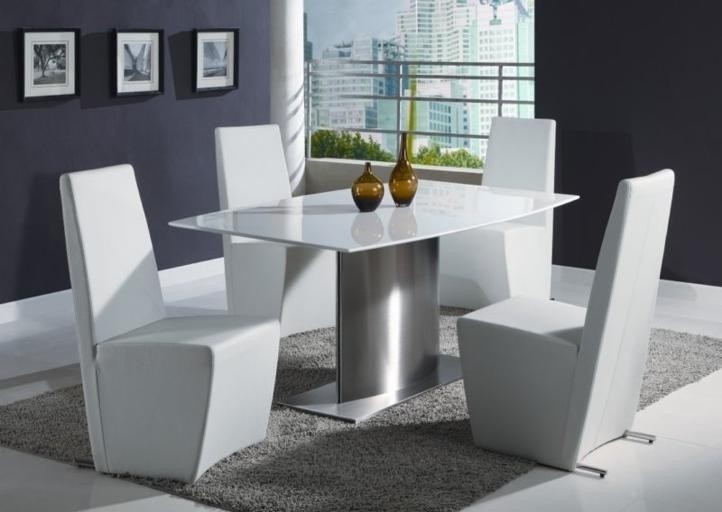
[435, 112, 559, 313]
[213, 124, 339, 340]
[453, 165, 677, 485]
[58, 162, 282, 487]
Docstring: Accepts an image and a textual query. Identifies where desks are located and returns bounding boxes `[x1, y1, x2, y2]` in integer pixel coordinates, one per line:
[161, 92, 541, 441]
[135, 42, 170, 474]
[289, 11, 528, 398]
[157, 177, 584, 432]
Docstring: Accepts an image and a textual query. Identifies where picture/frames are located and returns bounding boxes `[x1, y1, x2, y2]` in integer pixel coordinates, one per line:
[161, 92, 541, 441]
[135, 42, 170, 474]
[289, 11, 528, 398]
[191, 26, 242, 95]
[15, 27, 84, 104]
[112, 27, 167, 98]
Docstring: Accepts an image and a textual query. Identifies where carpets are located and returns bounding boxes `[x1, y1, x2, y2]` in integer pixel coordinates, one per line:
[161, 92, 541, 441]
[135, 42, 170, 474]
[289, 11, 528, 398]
[0, 303, 722, 512]
